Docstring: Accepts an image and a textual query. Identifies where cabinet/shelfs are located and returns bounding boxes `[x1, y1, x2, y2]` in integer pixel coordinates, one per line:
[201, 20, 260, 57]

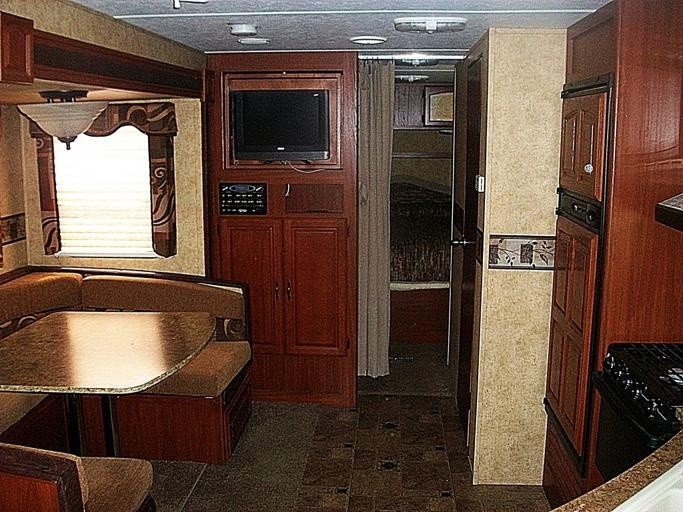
[203, 216, 360, 409]
[540, 0, 683, 512]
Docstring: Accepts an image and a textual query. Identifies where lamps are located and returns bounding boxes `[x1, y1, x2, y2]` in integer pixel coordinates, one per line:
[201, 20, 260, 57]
[18, 88, 109, 148]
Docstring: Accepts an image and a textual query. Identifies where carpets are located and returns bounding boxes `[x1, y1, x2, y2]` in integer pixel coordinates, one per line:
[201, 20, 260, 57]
[357, 343, 454, 398]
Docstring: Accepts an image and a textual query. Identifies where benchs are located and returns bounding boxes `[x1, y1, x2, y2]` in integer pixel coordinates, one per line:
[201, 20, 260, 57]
[0, 265, 253, 464]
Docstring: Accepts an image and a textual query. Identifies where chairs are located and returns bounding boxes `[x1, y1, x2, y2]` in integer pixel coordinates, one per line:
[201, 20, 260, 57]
[0, 440, 155, 511]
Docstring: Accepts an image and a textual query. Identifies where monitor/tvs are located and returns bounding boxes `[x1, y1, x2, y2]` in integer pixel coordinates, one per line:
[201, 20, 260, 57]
[233, 89, 330, 161]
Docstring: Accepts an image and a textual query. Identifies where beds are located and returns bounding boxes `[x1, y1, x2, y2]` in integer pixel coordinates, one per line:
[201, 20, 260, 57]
[390, 174, 451, 344]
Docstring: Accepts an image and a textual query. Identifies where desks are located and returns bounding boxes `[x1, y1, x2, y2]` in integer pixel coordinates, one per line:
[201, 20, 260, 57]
[0, 312, 218, 458]
